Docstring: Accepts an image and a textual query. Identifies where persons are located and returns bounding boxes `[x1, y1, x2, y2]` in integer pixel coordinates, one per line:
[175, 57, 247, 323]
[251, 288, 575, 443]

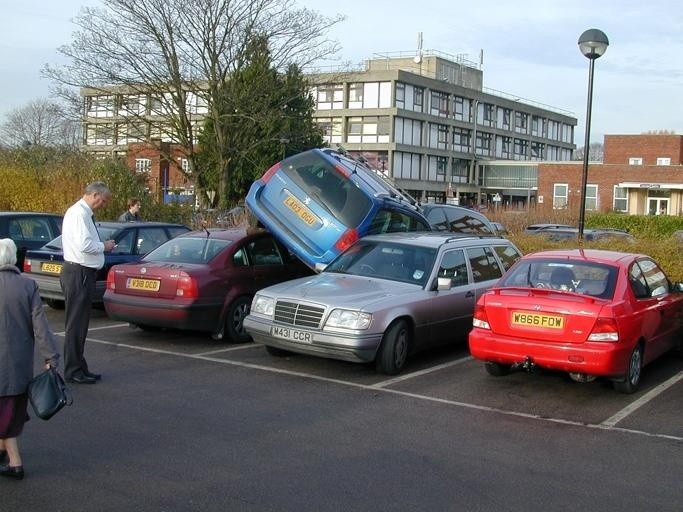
[117, 196, 144, 223]
[0, 236, 71, 479]
[60, 177, 115, 386]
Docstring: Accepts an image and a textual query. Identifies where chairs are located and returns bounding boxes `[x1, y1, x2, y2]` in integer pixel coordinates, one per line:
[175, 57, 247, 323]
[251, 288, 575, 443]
[116, 239, 157, 253]
[9, 224, 48, 241]
[551, 267, 575, 286]
[379, 255, 407, 275]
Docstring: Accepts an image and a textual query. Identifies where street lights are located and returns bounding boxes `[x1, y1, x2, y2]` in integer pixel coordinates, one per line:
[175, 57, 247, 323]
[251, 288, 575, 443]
[577, 26, 609, 243]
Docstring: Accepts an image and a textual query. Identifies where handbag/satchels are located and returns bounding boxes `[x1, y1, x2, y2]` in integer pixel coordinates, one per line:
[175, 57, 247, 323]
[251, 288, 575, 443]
[26, 368, 74, 419]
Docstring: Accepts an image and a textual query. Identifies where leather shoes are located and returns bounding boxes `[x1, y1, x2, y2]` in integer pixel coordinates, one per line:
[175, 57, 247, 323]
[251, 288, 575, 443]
[65, 370, 96, 384]
[0, 462, 24, 480]
[0, 450, 9, 464]
[86, 371, 101, 380]
